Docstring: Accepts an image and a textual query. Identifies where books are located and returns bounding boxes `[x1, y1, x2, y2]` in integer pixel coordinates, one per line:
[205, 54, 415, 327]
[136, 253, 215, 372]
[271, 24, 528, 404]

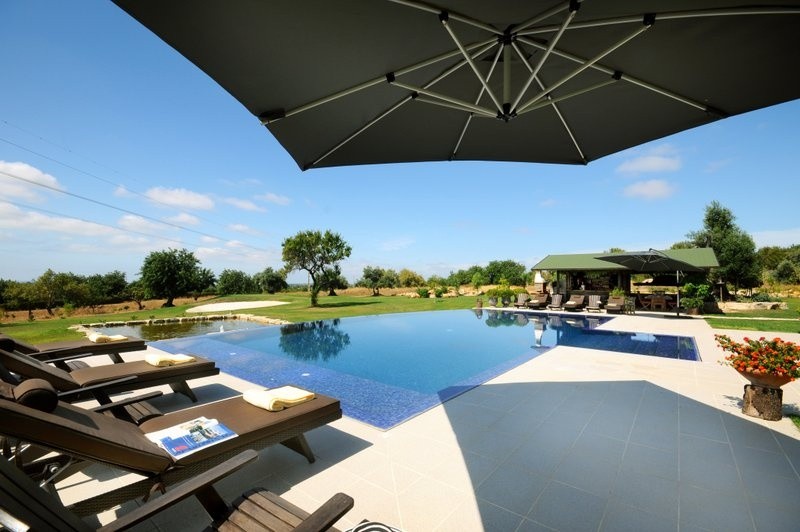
[143, 415, 240, 463]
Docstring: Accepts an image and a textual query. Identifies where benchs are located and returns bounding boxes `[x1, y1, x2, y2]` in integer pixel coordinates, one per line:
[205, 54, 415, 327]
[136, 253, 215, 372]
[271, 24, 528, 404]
[637, 291, 650, 311]
[668, 301, 677, 311]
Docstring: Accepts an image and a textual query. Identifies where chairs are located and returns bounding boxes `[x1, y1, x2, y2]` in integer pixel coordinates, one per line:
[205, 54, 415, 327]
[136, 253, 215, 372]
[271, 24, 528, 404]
[0, 382, 343, 496]
[0, 346, 221, 405]
[513, 293, 528, 309]
[586, 295, 602, 313]
[604, 295, 624, 314]
[622, 297, 636, 314]
[0, 334, 149, 372]
[545, 293, 562, 311]
[527, 293, 548, 308]
[563, 294, 585, 312]
[0, 448, 354, 532]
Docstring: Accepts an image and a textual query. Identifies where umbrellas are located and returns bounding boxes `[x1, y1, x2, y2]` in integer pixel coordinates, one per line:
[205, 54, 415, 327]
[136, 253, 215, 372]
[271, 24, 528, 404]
[116, 1, 799, 170]
[592, 251, 705, 318]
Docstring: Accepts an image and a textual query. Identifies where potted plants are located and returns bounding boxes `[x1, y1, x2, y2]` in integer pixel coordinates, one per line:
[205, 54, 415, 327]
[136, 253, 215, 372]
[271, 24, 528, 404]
[497, 290, 512, 307]
[680, 297, 704, 315]
[512, 288, 529, 303]
[486, 288, 501, 308]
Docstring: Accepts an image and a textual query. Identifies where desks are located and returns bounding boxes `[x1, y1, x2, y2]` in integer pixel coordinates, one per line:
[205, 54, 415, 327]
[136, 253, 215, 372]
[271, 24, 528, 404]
[650, 296, 666, 312]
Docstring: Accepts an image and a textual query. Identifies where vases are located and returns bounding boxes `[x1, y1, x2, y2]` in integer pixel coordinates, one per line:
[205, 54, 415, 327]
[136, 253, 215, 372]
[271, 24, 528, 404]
[735, 367, 791, 389]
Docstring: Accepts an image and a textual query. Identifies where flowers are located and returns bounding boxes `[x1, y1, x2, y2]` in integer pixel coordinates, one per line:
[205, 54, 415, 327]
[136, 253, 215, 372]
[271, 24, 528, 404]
[713, 332, 800, 381]
